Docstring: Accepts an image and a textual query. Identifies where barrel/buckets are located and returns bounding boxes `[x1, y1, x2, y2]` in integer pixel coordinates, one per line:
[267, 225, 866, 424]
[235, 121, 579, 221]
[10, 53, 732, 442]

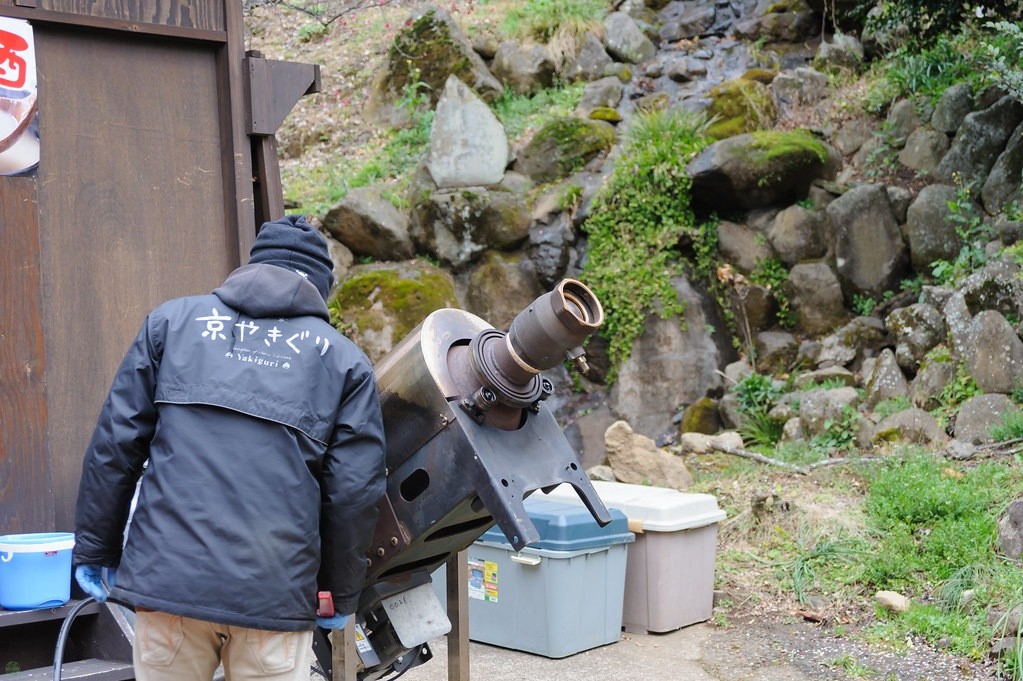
[0, 533, 76, 611]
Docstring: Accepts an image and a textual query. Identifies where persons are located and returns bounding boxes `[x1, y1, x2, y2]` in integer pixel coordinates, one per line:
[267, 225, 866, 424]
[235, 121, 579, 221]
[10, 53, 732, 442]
[70, 216, 388, 681]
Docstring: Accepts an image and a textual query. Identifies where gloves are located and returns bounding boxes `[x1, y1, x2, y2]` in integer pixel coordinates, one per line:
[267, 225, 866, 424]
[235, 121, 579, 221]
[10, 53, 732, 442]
[76, 564, 116, 602]
[316, 612, 349, 630]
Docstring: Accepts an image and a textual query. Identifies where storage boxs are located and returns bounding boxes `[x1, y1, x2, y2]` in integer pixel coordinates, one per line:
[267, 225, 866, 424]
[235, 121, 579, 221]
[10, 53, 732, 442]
[0, 532, 75, 609]
[430, 497, 636, 658]
[548, 479, 728, 635]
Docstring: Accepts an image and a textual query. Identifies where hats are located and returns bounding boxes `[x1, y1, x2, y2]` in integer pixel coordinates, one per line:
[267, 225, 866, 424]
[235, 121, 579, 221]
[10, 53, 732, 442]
[248, 215, 335, 297]
